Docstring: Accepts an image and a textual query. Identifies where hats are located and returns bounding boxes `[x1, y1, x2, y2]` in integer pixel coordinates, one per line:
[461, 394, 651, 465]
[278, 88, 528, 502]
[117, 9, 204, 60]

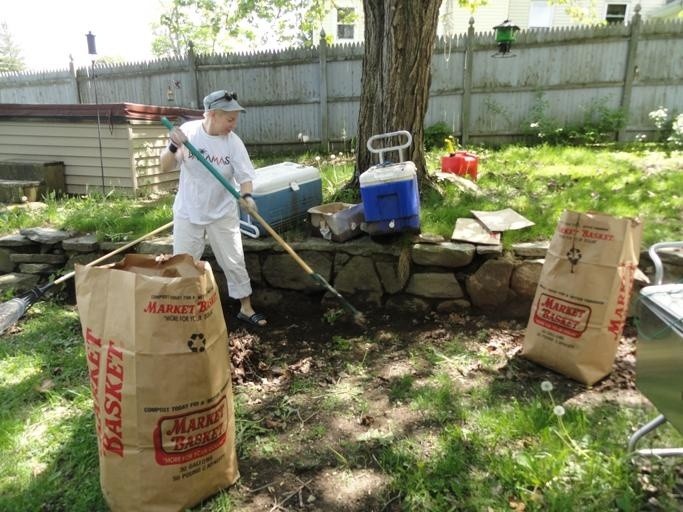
[204, 90, 246, 112]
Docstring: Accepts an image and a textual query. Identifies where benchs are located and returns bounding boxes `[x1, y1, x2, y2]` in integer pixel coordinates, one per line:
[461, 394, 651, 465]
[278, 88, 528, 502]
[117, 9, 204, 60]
[0, 159, 66, 204]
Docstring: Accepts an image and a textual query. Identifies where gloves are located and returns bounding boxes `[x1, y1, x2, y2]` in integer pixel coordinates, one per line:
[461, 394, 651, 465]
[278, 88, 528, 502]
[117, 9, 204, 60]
[241, 193, 257, 213]
[169, 126, 187, 153]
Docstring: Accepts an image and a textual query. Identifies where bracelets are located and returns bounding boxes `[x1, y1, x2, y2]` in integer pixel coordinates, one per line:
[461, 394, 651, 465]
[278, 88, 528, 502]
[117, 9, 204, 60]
[168, 141, 178, 153]
[243, 193, 252, 199]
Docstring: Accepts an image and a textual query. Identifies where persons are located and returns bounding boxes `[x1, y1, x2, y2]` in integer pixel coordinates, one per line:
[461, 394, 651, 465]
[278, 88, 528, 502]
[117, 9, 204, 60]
[159, 90, 268, 328]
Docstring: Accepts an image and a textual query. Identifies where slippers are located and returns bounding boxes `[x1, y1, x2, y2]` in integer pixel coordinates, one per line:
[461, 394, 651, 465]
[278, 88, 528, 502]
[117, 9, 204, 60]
[238, 312, 269, 328]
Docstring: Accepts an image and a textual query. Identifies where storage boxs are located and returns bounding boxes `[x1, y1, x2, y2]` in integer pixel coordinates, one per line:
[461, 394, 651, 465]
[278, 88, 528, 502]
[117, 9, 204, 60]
[232, 161, 323, 238]
[308, 202, 368, 243]
[358, 161, 419, 222]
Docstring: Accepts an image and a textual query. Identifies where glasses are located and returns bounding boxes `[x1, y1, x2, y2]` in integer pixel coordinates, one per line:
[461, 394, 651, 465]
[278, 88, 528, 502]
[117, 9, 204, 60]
[209, 92, 237, 108]
